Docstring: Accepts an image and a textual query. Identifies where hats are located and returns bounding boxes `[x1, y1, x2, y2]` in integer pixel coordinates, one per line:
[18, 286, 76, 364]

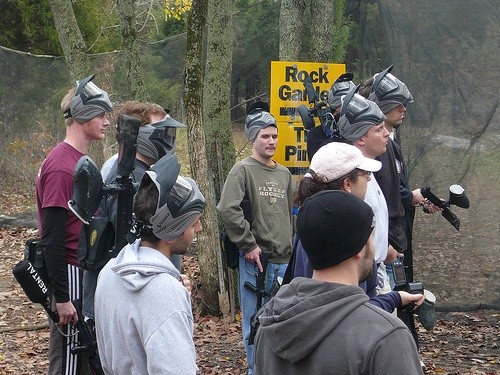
[295, 190, 375, 270]
[309, 142, 383, 183]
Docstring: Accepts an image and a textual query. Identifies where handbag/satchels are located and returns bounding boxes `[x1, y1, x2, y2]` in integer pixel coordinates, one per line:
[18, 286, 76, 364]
[78, 217, 115, 273]
[220, 195, 251, 270]
[24, 239, 46, 277]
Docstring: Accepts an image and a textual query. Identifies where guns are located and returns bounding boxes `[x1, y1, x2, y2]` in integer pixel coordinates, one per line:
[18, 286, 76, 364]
[393, 281, 437, 351]
[244, 253, 284, 346]
[12, 259, 105, 375]
[297, 76, 340, 139]
[68, 112, 142, 258]
[421, 184, 470, 231]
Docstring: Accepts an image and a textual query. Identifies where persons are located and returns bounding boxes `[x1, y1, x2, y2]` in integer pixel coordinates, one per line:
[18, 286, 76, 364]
[216, 101, 296, 375]
[95, 145, 207, 375]
[252, 189, 424, 375]
[35, 74, 113, 375]
[294, 63, 446, 313]
[82, 101, 187, 320]
